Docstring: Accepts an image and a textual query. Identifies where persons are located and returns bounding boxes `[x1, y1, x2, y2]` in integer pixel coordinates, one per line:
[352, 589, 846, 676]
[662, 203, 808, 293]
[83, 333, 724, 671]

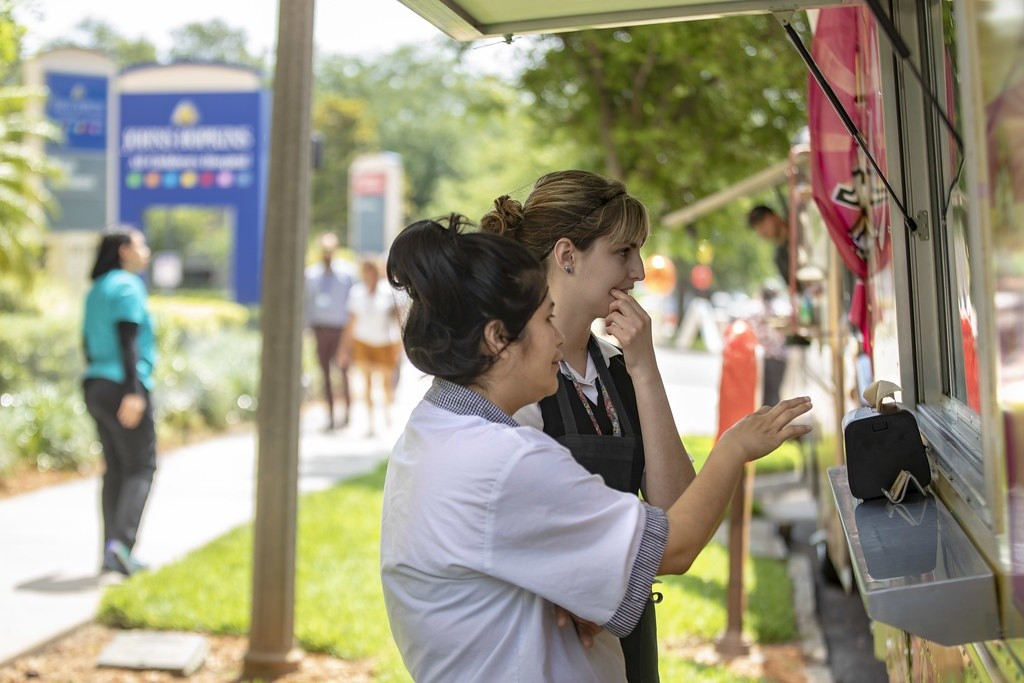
[334, 255, 412, 429]
[742, 288, 791, 407]
[302, 232, 363, 434]
[474, 171, 696, 683]
[744, 203, 803, 294]
[385, 210, 812, 683]
[80, 222, 161, 578]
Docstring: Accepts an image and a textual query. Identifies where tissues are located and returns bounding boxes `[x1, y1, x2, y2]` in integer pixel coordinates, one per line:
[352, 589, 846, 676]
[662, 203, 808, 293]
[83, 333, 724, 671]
[842, 378, 937, 506]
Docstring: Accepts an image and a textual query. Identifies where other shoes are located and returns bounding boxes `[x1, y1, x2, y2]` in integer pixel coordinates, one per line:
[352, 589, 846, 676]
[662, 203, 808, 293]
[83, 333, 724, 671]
[102, 538, 131, 576]
[101, 560, 144, 579]
